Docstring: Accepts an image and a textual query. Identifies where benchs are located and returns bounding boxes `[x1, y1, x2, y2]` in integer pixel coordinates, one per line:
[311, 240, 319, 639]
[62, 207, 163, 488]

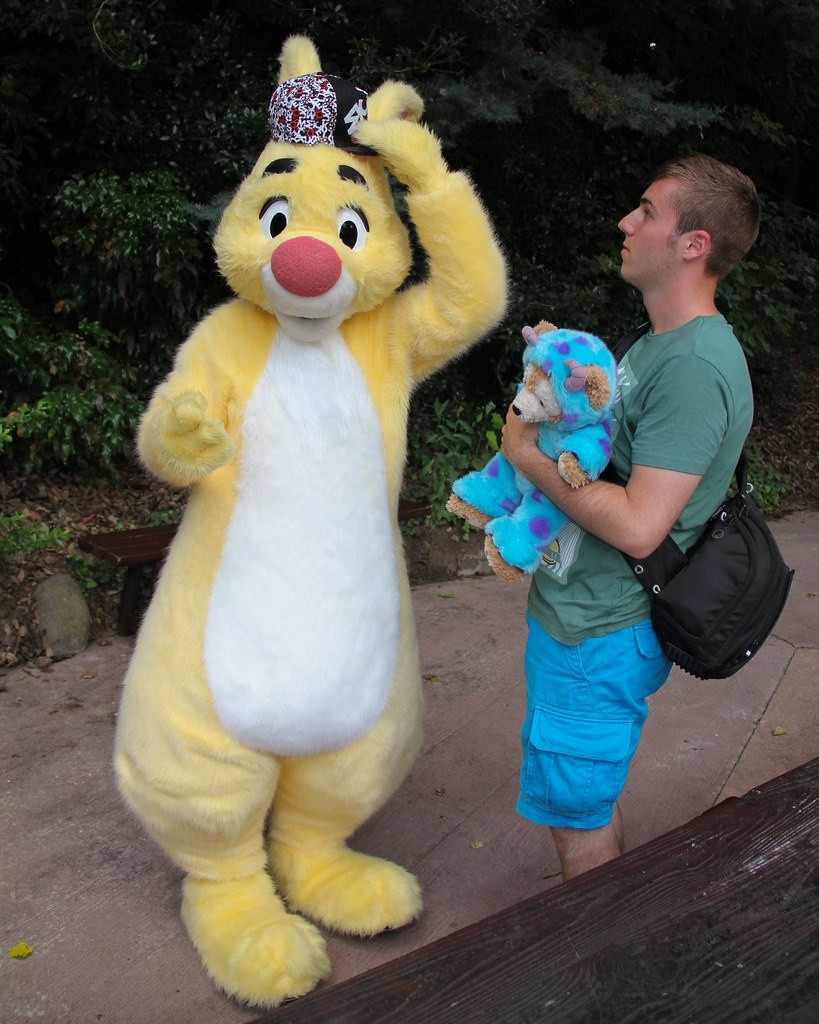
[244, 754, 819, 1024]
[78, 495, 434, 636]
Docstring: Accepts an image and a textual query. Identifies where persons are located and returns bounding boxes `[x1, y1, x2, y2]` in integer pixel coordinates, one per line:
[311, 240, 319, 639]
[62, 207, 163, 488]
[501, 154, 761, 877]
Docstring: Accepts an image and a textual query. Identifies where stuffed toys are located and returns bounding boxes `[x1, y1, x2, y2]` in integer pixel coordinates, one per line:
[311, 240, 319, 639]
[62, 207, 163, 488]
[447, 324, 615, 580]
[113, 37, 514, 1007]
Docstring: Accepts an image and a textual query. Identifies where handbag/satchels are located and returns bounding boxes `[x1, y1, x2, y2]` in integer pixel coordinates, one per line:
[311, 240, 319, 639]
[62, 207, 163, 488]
[647, 490, 795, 679]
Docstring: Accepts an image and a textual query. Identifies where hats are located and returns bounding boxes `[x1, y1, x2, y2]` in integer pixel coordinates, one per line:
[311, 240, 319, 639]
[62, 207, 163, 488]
[265, 72, 379, 157]
